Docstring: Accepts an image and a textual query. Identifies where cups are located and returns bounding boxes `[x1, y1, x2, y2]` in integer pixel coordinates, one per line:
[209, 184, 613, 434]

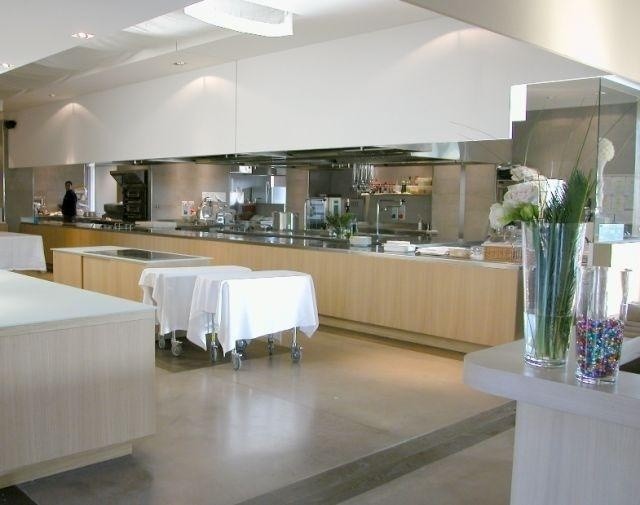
[573, 265, 629, 387]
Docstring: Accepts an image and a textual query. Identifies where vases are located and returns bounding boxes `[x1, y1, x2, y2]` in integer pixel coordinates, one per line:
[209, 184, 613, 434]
[523, 220, 586, 368]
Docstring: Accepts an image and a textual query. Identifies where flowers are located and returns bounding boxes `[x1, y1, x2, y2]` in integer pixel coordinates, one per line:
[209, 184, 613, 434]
[453, 80, 636, 357]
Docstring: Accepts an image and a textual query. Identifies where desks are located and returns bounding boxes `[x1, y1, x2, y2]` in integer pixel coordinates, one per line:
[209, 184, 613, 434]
[51, 244, 214, 336]
[0, 230, 47, 271]
[0, 269, 154, 489]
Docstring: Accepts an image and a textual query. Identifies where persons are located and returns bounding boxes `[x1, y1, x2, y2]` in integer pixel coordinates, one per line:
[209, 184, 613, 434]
[56, 179, 78, 224]
